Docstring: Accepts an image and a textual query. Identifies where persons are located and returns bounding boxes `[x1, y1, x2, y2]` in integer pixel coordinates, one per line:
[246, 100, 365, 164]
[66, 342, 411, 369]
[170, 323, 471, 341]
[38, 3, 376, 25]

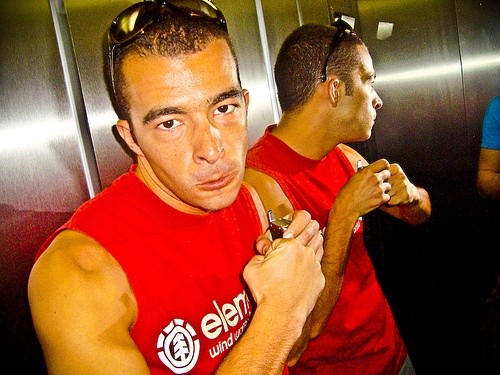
[248, 13, 432, 375]
[27, 0, 326, 375]
[476, 94, 500, 205]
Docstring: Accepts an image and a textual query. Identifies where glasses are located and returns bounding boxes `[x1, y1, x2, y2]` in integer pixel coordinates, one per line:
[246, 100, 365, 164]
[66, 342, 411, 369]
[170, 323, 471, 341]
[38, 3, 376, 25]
[321, 19, 355, 83]
[107, 0, 229, 104]
